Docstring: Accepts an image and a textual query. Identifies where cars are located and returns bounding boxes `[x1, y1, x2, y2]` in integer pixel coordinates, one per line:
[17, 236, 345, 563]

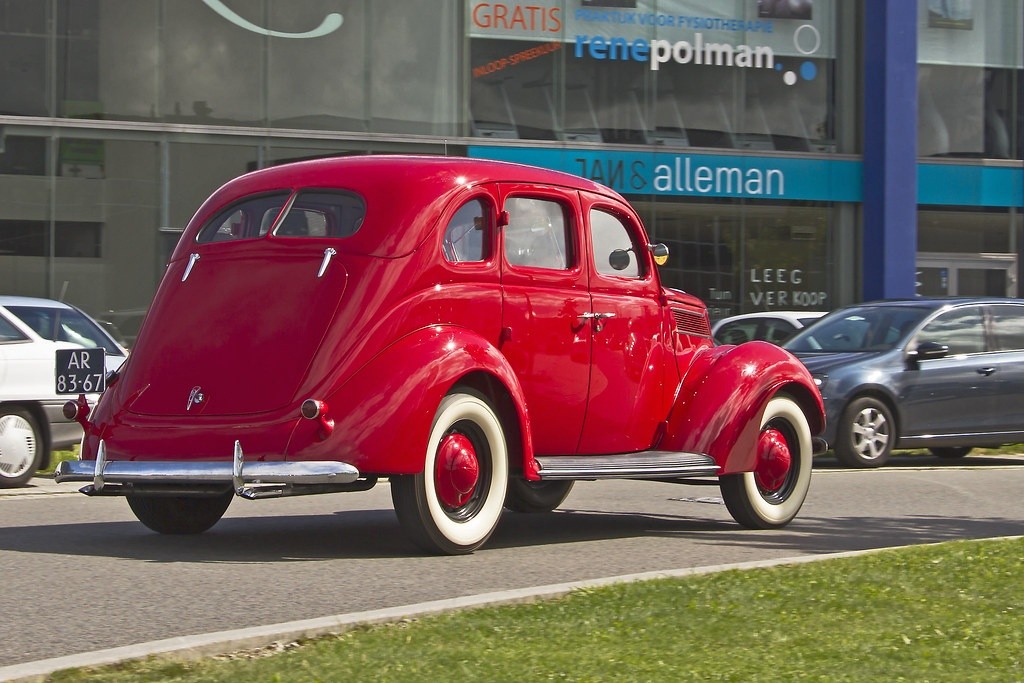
[777, 295, 1024, 469]
[52, 154, 828, 556]
[711, 311, 903, 352]
[0, 294, 128, 489]
